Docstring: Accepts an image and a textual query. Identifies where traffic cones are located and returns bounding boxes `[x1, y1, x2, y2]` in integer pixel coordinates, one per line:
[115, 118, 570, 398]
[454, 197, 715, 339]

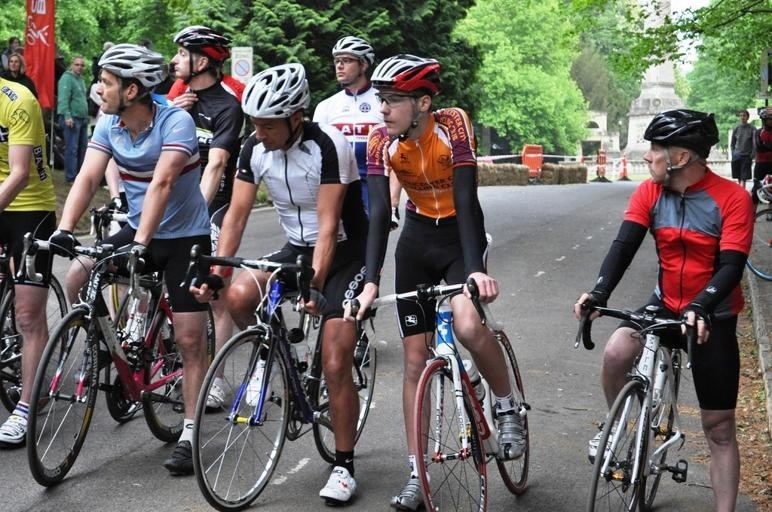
[587, 148, 613, 182]
[619, 155, 633, 182]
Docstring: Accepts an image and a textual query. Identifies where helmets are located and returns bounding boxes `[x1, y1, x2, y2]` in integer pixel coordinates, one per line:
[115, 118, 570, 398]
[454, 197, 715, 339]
[644, 110, 718, 158]
[332, 36, 375, 66]
[760, 107, 772, 119]
[370, 53, 442, 95]
[173, 25, 232, 64]
[97, 43, 168, 88]
[241, 63, 310, 119]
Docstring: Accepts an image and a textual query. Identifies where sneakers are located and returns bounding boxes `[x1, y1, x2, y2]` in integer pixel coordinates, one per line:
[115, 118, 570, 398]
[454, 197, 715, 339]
[391, 476, 423, 511]
[0, 415, 28, 444]
[319, 466, 356, 501]
[245, 360, 273, 406]
[205, 377, 225, 407]
[162, 441, 194, 471]
[497, 400, 528, 459]
[588, 415, 627, 459]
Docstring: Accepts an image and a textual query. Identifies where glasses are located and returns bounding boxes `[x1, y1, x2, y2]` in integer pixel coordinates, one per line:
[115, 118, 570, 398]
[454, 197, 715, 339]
[75, 64, 84, 67]
[377, 96, 406, 105]
[334, 57, 354, 65]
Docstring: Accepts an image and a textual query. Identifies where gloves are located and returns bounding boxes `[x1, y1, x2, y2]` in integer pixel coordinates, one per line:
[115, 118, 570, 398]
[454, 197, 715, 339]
[49, 230, 80, 260]
[113, 241, 151, 275]
[190, 274, 224, 301]
[300, 288, 328, 312]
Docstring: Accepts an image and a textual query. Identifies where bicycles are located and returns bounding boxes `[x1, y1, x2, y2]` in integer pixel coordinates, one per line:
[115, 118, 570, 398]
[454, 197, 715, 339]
[745, 176, 772, 285]
[349, 277, 532, 507]
[179, 244, 377, 507]
[572, 295, 696, 507]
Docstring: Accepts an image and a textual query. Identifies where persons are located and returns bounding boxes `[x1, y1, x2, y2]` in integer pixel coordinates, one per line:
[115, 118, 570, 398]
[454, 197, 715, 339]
[574, 108, 754, 512]
[180, 64, 379, 501]
[170, 23, 244, 413]
[343, 53, 529, 512]
[1, 77, 57, 442]
[751, 107, 772, 222]
[731, 110, 757, 189]
[49, 43, 212, 475]
[57, 58, 103, 184]
[1, 37, 38, 100]
[312, 36, 403, 364]
[164, 62, 247, 141]
